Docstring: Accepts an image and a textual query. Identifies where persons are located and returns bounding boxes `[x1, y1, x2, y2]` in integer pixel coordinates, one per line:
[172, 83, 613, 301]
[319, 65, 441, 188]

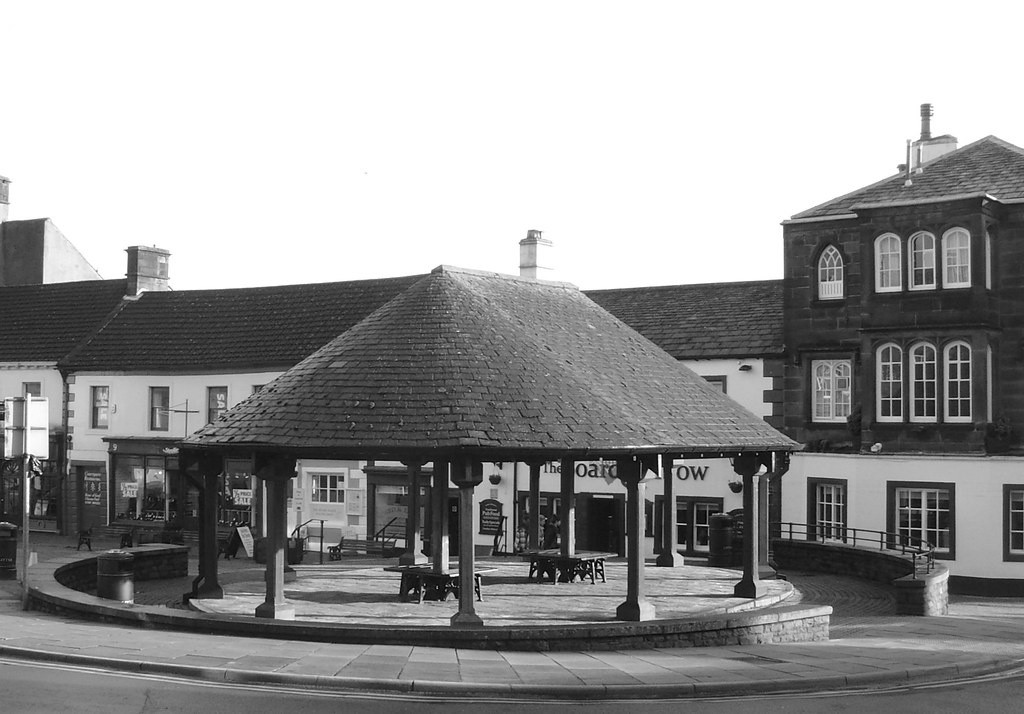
[539, 515, 558, 549]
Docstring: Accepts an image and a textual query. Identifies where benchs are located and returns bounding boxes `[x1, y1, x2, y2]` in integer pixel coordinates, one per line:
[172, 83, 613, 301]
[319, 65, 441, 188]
[327, 536, 396, 560]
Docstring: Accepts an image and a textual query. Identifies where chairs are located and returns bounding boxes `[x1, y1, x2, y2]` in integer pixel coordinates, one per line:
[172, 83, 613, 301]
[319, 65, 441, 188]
[77, 522, 245, 560]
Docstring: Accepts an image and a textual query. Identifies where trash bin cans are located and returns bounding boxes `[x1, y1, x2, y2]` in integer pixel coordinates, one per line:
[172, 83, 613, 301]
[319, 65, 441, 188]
[96, 550, 136, 604]
[708, 513, 734, 567]
[0, 522, 18, 580]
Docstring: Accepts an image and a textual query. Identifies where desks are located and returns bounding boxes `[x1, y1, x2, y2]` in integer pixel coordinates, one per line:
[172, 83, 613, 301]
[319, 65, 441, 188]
[517, 548, 619, 585]
[382, 564, 498, 604]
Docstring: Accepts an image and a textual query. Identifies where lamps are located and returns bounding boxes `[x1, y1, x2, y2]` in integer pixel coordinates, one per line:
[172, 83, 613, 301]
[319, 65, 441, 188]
[738, 365, 752, 371]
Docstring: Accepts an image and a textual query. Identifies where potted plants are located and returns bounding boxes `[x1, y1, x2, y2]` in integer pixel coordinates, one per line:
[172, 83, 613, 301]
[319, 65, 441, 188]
[728, 479, 742, 493]
[489, 473, 501, 484]
